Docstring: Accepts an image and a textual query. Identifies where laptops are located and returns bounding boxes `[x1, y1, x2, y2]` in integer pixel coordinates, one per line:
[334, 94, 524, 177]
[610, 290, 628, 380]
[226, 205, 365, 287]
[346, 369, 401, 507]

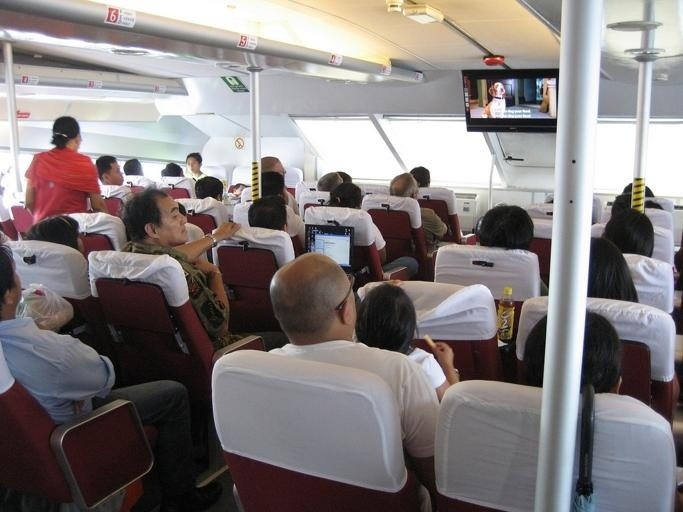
[303, 223, 354, 277]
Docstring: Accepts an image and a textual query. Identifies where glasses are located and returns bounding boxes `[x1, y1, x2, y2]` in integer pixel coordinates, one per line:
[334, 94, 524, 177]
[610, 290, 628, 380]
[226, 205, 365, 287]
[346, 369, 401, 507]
[336, 275, 355, 310]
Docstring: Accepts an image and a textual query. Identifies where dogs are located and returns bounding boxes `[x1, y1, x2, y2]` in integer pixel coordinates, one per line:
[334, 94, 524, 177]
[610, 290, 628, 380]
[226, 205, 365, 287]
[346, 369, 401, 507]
[482, 82, 508, 119]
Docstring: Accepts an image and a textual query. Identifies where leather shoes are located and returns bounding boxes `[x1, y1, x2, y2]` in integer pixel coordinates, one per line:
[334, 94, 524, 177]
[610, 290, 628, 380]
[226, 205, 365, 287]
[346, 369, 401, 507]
[160, 480, 222, 512]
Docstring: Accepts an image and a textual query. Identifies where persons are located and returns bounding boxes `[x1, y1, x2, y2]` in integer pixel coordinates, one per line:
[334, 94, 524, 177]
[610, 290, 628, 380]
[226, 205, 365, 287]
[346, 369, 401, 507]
[1, 115, 683, 512]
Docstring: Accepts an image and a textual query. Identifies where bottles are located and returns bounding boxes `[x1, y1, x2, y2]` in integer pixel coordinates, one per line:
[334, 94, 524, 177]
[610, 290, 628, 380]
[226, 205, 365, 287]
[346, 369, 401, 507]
[496, 286, 515, 341]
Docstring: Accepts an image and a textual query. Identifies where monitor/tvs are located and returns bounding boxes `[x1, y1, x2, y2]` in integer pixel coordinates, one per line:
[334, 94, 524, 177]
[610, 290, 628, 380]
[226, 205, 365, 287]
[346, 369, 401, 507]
[461, 67, 560, 132]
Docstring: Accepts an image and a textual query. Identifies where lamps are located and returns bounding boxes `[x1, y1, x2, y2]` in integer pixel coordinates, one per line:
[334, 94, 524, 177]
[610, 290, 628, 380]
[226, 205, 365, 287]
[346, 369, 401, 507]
[402, 3, 444, 24]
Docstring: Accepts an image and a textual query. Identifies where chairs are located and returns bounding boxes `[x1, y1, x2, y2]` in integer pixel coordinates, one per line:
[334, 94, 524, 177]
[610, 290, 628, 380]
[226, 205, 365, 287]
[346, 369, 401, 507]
[515, 296, 678, 429]
[351, 281, 499, 386]
[0, 334, 155, 510]
[1, 170, 682, 330]
[86, 249, 268, 399]
[433, 379, 678, 509]
[209, 349, 431, 510]
